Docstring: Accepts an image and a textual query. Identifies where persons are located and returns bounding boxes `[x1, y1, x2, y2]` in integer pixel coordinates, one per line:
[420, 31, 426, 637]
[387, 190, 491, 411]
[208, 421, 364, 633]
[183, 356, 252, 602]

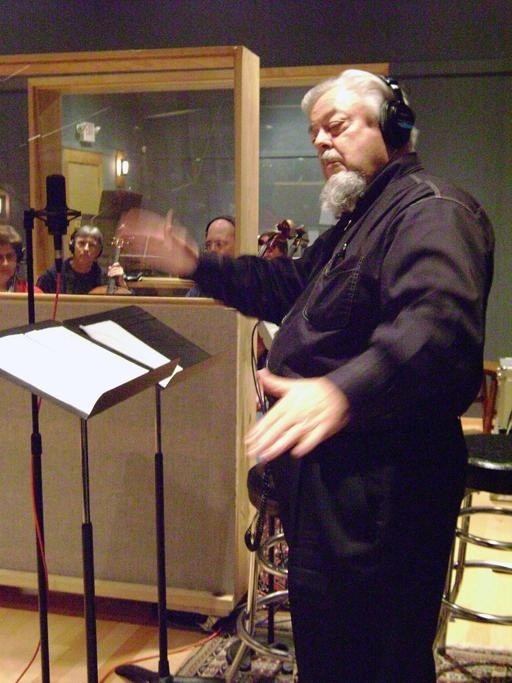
[0, 223, 45, 294]
[189, 213, 234, 296]
[36, 225, 133, 295]
[256, 227, 295, 260]
[114, 66, 497, 683]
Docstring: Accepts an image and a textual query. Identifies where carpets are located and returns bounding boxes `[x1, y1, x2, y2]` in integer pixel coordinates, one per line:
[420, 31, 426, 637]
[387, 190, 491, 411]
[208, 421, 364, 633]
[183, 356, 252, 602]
[170, 623, 512, 679]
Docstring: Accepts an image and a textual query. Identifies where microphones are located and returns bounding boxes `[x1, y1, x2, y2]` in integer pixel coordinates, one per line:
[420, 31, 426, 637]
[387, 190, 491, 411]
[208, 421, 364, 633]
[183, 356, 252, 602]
[46, 175, 67, 268]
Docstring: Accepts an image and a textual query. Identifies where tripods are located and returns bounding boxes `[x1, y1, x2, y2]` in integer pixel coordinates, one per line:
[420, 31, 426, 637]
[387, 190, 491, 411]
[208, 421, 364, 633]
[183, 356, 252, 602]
[113, 383, 226, 683]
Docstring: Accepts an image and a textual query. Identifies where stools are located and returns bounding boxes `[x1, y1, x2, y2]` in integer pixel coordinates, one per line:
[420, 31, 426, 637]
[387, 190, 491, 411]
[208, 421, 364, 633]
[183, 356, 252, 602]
[222, 459, 304, 682]
[435, 434, 512, 683]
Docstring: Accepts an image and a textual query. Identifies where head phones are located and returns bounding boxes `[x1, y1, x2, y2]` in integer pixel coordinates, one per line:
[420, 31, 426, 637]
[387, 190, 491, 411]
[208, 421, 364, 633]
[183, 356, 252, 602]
[16, 243, 24, 261]
[69, 227, 103, 259]
[379, 73, 414, 149]
